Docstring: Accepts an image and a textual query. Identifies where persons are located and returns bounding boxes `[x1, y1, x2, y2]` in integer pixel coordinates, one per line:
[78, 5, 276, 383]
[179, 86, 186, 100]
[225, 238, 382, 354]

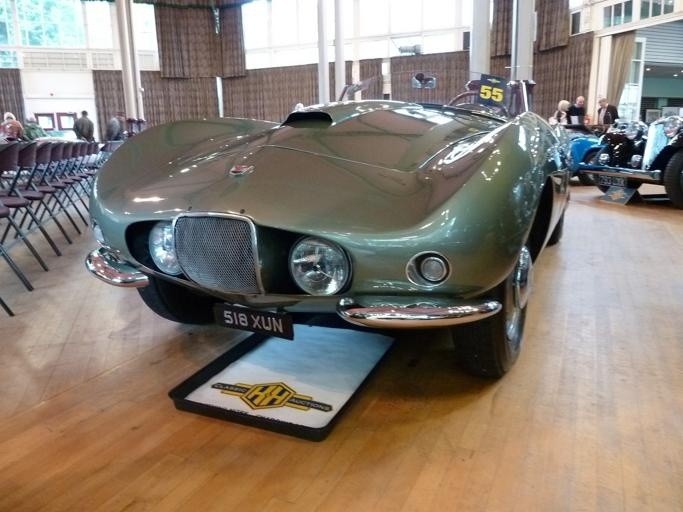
[72, 111, 94, 141]
[553, 100, 570, 124]
[20, 115, 50, 142]
[596, 97, 620, 125]
[0, 112, 23, 145]
[105, 111, 120, 141]
[566, 96, 585, 123]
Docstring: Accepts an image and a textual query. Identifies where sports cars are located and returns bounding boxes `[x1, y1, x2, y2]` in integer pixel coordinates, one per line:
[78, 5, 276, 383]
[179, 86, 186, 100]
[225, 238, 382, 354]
[82, 67, 571, 380]
[553, 112, 607, 184]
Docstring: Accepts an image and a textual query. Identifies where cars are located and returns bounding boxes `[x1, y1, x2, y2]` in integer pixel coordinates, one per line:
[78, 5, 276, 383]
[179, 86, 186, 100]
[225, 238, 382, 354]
[577, 115, 682, 208]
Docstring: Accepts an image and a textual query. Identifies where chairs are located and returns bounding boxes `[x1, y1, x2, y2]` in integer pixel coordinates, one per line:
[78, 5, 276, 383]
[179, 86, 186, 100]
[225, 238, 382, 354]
[0, 138, 122, 317]
